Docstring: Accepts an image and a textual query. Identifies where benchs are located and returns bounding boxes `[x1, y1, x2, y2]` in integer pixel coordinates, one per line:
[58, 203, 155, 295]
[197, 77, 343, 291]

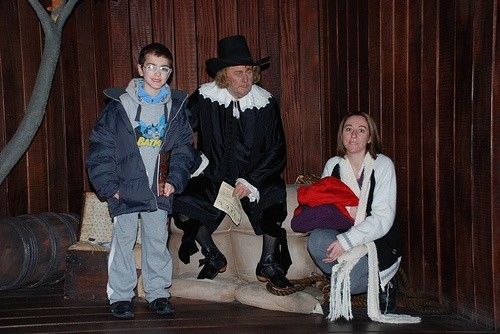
[63, 185, 403, 315]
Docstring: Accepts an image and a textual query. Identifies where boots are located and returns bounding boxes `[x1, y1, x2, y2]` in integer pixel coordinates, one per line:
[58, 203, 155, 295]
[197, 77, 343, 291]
[256, 233, 291, 291]
[197, 237, 227, 280]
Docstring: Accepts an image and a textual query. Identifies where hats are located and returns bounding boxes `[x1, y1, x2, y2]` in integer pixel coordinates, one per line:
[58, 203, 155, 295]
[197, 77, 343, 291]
[205, 36, 270, 79]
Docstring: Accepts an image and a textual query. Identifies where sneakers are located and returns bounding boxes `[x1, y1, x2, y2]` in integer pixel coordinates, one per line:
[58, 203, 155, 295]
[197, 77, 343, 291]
[111, 301, 134, 318]
[148, 298, 174, 314]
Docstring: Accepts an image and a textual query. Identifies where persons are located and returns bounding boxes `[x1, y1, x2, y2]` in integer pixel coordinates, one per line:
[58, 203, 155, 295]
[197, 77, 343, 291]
[87, 43, 196, 318]
[307, 111, 402, 313]
[173, 34, 294, 289]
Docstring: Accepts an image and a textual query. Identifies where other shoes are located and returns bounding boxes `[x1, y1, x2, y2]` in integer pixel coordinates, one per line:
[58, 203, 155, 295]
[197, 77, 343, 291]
[379, 277, 398, 315]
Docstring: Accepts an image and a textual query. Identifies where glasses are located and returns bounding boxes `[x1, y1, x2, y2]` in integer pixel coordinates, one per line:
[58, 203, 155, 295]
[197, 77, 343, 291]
[143, 65, 170, 74]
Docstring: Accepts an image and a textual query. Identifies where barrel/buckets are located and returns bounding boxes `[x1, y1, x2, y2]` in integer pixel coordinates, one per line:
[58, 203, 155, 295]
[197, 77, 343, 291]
[0, 212, 81, 291]
